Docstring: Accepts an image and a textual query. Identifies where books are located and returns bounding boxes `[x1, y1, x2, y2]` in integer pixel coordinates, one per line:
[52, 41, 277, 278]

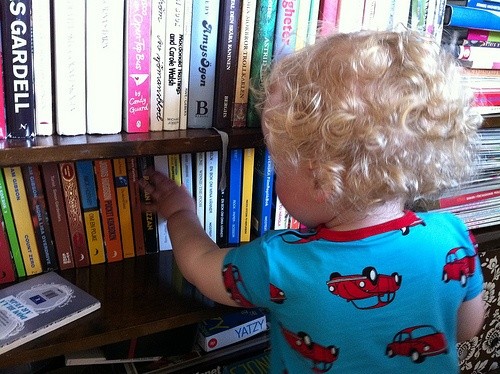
[0, 0, 500, 291]
[65, 356, 163, 367]
[0, 269, 102, 356]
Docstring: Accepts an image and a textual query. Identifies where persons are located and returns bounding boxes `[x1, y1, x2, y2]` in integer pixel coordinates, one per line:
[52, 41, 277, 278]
[133, 25, 486, 374]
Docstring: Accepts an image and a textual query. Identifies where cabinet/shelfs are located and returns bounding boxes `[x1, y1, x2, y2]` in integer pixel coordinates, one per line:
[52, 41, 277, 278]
[0, 115, 500, 374]
